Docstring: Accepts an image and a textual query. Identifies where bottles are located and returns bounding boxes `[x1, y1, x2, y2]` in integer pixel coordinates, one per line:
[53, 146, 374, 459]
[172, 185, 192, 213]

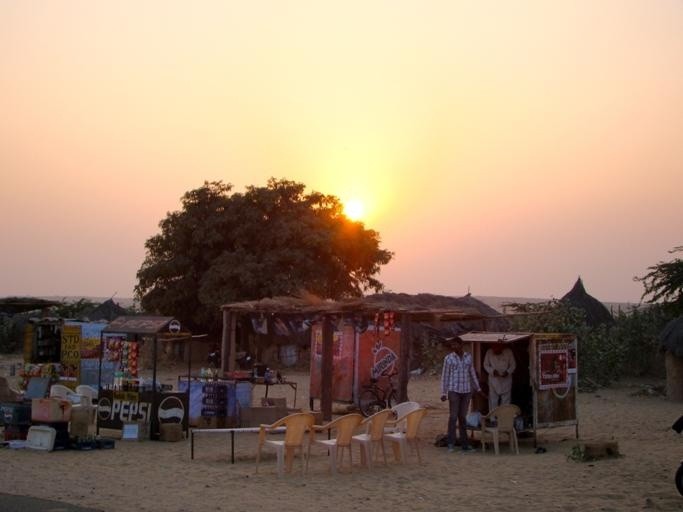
[201, 367, 212, 383]
[264, 367, 270, 384]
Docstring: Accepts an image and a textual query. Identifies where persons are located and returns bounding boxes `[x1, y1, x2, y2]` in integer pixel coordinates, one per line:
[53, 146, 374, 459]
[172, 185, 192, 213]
[438, 336, 487, 452]
[483, 342, 518, 412]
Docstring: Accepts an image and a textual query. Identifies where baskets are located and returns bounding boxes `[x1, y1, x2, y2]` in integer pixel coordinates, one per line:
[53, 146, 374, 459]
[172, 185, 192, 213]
[159, 422, 183, 442]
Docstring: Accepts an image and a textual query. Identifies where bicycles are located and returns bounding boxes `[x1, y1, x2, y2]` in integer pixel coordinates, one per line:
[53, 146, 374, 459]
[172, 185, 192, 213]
[359, 369, 410, 418]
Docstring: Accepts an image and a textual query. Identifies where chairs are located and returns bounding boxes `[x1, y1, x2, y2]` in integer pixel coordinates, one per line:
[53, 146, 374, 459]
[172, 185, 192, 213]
[254, 402, 523, 480]
[46, 382, 100, 436]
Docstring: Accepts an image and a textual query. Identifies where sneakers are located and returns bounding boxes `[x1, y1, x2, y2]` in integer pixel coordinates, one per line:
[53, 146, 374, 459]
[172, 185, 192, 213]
[447, 443, 456, 453]
[459, 445, 475, 452]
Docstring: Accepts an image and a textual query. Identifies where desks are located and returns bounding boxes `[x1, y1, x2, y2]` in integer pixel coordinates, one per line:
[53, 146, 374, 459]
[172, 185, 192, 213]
[187, 424, 331, 464]
